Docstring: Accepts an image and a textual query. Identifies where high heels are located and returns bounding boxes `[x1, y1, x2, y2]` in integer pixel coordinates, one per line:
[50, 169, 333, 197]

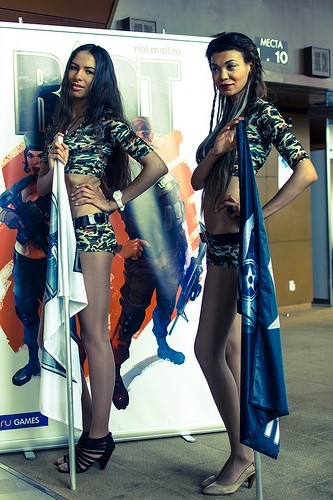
[200, 461, 256, 487]
[202, 462, 256, 495]
[55, 432, 91, 466]
[57, 432, 116, 473]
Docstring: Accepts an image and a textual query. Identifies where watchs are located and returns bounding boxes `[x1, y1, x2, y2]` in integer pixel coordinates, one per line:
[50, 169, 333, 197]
[112, 190, 125, 211]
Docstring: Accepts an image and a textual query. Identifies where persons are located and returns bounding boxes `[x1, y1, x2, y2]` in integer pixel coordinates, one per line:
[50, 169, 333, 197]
[191, 32, 318, 495]
[37, 44, 169, 474]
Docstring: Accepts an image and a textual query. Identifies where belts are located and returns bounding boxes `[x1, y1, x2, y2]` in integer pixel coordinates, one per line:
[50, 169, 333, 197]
[72, 213, 108, 225]
[203, 231, 240, 243]
